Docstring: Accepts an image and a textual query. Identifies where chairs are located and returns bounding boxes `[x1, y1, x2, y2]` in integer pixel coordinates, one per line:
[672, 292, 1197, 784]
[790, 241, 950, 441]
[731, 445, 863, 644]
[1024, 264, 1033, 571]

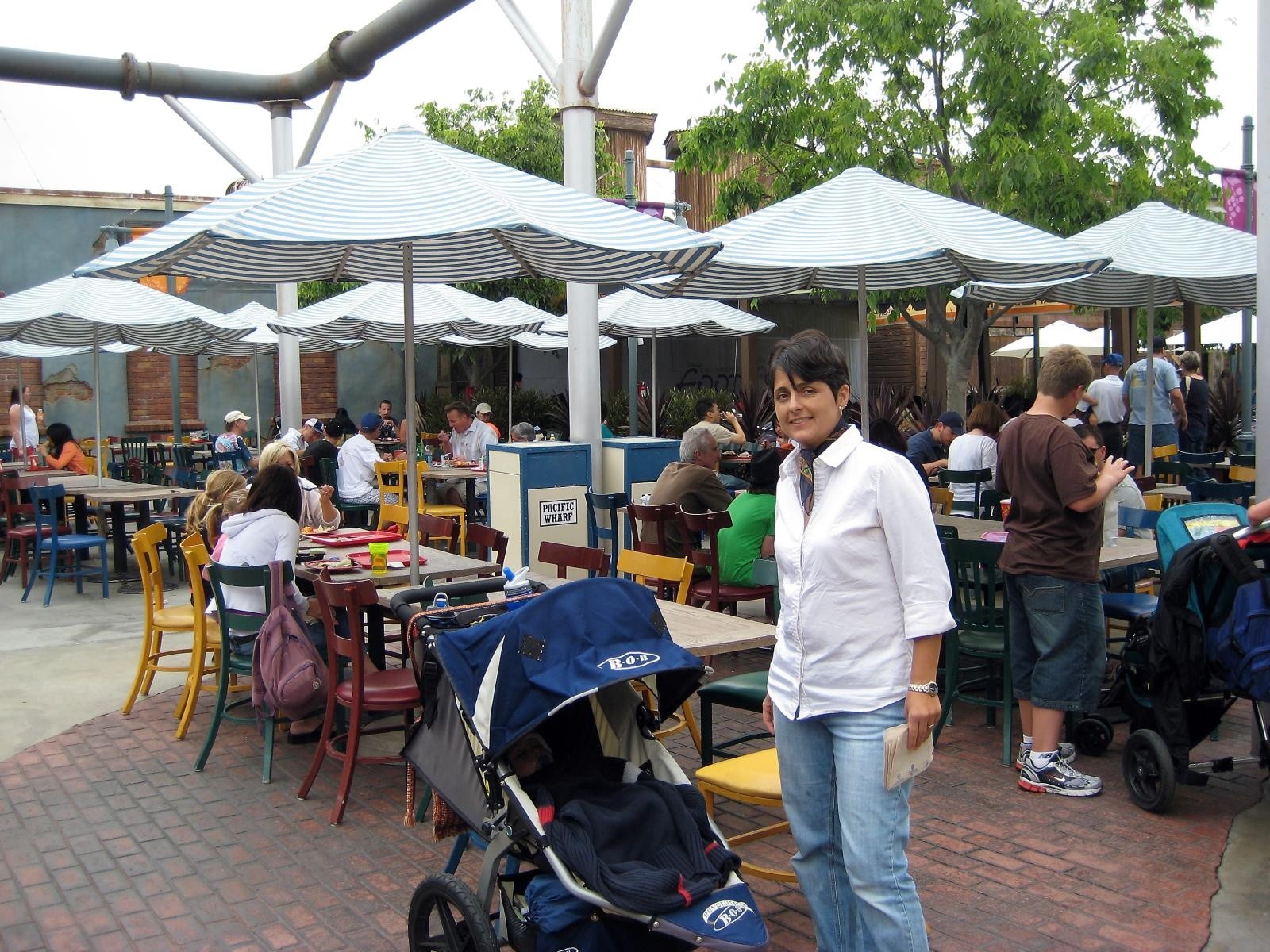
[0, 435, 1016, 884]
[1102, 446, 1257, 695]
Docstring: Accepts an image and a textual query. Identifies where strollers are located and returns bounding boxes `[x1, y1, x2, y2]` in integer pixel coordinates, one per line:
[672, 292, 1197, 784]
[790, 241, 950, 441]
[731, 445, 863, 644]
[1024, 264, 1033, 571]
[390, 573, 770, 952]
[1071, 501, 1270, 816]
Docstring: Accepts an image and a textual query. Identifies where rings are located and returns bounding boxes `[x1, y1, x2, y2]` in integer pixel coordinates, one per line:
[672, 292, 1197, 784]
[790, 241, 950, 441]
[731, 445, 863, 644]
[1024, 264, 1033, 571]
[928, 723, 935, 729]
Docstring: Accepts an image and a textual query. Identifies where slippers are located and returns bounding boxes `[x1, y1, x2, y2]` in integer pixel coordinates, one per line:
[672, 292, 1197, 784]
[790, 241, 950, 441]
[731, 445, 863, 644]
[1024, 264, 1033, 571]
[288, 722, 337, 744]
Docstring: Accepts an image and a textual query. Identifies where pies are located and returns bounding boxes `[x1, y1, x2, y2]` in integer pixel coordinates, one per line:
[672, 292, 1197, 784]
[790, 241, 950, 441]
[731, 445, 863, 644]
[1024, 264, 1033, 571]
[318, 559, 355, 567]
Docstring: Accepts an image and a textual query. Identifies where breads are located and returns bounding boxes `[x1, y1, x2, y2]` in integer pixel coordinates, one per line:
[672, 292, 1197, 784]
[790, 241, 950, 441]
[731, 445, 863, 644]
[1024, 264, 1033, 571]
[303, 525, 313, 533]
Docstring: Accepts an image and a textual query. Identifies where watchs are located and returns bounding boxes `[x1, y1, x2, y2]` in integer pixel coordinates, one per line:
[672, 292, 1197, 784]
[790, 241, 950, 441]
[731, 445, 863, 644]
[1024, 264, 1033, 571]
[908, 681, 939, 697]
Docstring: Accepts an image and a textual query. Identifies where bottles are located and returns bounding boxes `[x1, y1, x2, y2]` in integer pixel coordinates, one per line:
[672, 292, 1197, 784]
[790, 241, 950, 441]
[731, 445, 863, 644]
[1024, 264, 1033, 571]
[720, 413, 742, 421]
[391, 425, 396, 438]
[424, 449, 432, 466]
[1103, 491, 1119, 547]
[38, 409, 44, 430]
[550, 434, 555, 440]
[503, 566, 533, 611]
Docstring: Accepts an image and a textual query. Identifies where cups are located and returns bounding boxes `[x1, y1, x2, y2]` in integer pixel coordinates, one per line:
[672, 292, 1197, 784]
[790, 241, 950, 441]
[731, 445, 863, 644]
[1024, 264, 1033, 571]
[1000, 499, 1011, 523]
[30, 456, 38, 467]
[369, 542, 389, 574]
[394, 450, 404, 459]
[0, 459, 2, 472]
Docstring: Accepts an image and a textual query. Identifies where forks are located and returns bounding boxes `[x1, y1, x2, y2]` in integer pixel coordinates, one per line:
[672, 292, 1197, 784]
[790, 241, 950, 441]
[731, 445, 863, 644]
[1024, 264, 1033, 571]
[302, 487, 321, 491]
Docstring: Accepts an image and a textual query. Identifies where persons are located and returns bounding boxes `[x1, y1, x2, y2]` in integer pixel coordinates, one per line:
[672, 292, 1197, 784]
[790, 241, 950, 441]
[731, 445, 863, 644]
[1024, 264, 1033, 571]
[9, 330, 1269, 797]
[762, 331, 959, 952]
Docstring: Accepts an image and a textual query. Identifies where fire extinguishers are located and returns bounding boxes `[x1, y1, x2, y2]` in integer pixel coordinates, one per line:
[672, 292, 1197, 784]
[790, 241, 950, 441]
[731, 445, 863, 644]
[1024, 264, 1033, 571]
[637, 379, 650, 412]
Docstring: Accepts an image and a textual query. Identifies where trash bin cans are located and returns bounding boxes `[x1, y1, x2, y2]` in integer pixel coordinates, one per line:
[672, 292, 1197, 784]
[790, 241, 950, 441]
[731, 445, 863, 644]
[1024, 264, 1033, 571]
[486, 439, 595, 587]
[599, 435, 683, 580]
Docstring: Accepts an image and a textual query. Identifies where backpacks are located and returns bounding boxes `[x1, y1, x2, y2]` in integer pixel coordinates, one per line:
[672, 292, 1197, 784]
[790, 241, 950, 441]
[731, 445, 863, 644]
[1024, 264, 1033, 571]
[252, 560, 328, 719]
[1202, 533, 1270, 702]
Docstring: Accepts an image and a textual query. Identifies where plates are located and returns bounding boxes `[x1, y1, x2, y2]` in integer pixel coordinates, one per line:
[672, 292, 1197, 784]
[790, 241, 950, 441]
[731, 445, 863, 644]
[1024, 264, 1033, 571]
[721, 453, 737, 457]
[296, 553, 327, 563]
[301, 528, 338, 534]
[305, 560, 362, 573]
[737, 457, 751, 459]
[472, 469, 486, 471]
[455, 464, 478, 467]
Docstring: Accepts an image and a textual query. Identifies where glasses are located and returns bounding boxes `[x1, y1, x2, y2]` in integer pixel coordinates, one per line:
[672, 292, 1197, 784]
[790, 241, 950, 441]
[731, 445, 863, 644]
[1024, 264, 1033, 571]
[1090, 447, 1102, 454]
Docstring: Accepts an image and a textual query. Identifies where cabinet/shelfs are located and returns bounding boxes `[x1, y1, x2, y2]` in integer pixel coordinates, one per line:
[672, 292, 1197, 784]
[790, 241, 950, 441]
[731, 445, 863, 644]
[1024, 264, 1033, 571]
[485, 444, 591, 583]
[601, 437, 681, 581]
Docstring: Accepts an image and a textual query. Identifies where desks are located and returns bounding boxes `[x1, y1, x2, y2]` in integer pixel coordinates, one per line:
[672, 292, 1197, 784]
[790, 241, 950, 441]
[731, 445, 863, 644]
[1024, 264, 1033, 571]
[1144, 481, 1191, 502]
[5, 442, 1157, 738]
[1174, 456, 1232, 484]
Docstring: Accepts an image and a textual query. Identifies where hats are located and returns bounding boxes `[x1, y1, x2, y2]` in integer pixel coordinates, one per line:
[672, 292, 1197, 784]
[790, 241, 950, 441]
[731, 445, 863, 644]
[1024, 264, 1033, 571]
[1154, 336, 1166, 347]
[361, 413, 382, 431]
[305, 418, 325, 433]
[326, 420, 343, 436]
[476, 403, 493, 416]
[1101, 354, 1124, 366]
[224, 410, 252, 424]
[938, 411, 965, 435]
[745, 447, 783, 486]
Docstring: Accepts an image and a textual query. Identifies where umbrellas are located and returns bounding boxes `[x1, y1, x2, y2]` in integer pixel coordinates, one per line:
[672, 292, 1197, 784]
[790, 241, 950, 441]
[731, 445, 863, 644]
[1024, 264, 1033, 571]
[0, 132, 1257, 583]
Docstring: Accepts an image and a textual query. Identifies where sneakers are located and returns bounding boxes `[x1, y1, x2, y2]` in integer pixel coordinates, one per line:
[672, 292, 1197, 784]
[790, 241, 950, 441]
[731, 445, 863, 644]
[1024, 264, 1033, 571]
[1016, 741, 1076, 770]
[1017, 753, 1103, 796]
